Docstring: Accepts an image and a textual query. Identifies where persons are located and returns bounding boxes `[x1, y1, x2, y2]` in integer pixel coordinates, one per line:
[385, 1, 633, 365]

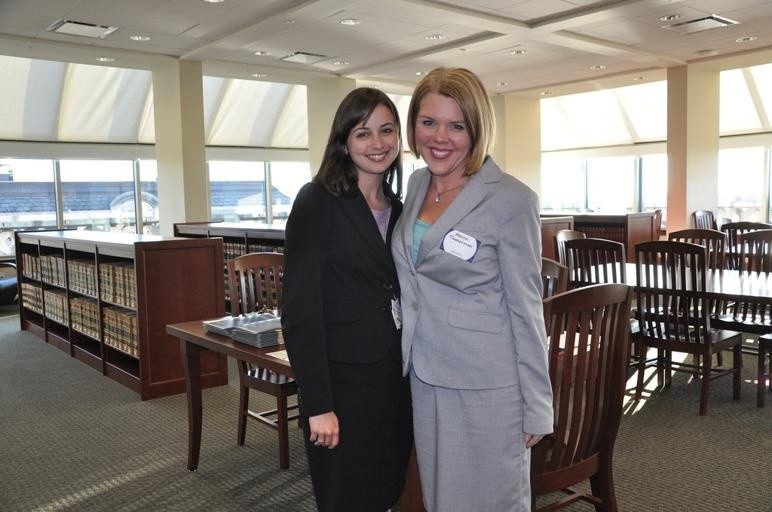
[390, 65, 561, 510]
[279, 86, 408, 512]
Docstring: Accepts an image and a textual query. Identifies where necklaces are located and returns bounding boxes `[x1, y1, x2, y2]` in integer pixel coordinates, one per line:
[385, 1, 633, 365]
[431, 179, 465, 204]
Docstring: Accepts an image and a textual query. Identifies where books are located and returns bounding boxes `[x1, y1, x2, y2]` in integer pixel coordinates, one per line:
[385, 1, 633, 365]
[575, 221, 626, 264]
[199, 240, 284, 348]
[20, 248, 142, 360]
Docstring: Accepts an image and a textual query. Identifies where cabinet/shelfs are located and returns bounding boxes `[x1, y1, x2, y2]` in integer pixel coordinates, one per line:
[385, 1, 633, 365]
[13, 227, 228, 400]
